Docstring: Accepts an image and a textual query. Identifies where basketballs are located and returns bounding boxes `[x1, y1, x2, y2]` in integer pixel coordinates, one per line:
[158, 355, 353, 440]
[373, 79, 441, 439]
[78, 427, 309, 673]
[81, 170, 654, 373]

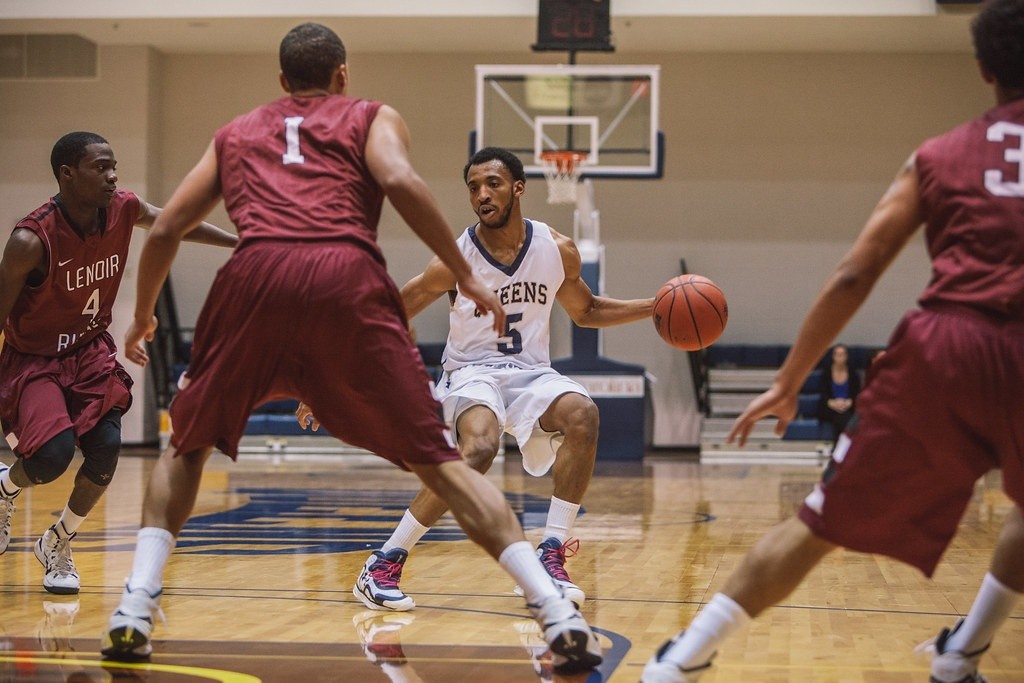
[653, 274, 728, 352]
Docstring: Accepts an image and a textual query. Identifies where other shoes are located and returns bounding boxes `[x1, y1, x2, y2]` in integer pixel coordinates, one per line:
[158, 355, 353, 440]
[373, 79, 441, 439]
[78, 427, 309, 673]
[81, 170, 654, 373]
[639, 639, 712, 683]
[100, 578, 167, 660]
[914, 618, 991, 683]
[526, 597, 603, 674]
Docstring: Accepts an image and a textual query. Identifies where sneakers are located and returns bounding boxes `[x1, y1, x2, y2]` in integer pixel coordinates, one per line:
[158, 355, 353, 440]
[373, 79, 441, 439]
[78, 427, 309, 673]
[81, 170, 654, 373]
[34, 596, 80, 659]
[352, 612, 416, 666]
[33, 523, 81, 596]
[352, 548, 415, 611]
[514, 537, 586, 611]
[0, 461, 22, 556]
[513, 622, 558, 680]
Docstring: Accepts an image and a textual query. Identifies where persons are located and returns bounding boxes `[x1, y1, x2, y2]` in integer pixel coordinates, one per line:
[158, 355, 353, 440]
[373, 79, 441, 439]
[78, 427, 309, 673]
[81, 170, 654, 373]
[293, 147, 655, 613]
[638, 1, 1024, 683]
[819, 342, 862, 444]
[868, 346, 888, 367]
[0, 132, 242, 595]
[101, 22, 604, 674]
